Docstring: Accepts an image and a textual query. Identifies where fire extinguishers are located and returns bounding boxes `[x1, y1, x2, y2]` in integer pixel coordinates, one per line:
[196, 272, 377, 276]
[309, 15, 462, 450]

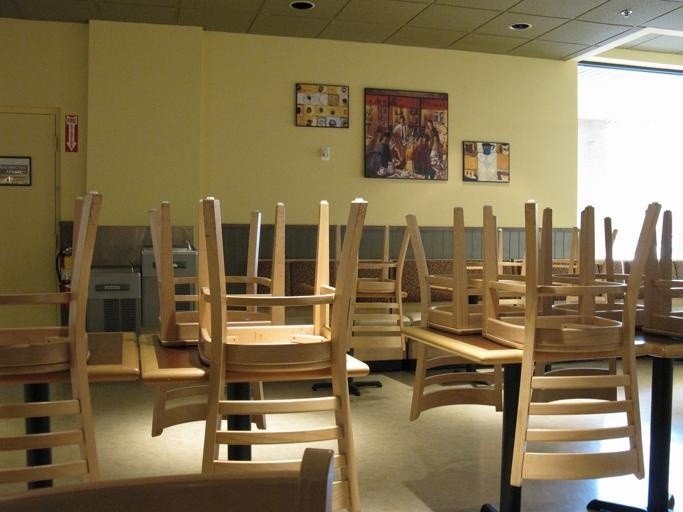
[55, 241, 73, 310]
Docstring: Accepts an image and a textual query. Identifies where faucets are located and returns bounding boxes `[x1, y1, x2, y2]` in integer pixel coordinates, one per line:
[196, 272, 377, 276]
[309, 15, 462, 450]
[127, 261, 136, 272]
[183, 239, 192, 250]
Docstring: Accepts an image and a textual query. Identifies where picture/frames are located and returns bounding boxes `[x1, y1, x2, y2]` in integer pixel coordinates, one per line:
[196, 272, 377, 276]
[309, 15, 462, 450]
[460, 141, 511, 184]
[294, 82, 350, 129]
[363, 87, 447, 180]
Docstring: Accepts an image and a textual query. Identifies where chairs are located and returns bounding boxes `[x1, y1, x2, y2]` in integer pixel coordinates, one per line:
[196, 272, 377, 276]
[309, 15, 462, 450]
[1, 194, 682, 512]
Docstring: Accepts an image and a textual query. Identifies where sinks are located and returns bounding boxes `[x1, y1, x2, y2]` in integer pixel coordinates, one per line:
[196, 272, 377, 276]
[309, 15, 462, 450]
[87, 268, 141, 300]
[143, 247, 196, 253]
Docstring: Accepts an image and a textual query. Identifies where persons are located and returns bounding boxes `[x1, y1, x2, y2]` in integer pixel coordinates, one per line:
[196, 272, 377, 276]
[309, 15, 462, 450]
[366, 114, 444, 180]
